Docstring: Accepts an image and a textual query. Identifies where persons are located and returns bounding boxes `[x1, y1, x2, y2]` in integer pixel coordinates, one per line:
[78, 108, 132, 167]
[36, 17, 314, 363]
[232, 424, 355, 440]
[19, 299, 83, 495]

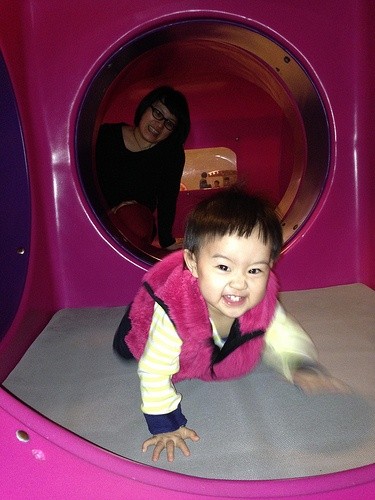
[112, 188, 352, 462]
[91, 87, 191, 250]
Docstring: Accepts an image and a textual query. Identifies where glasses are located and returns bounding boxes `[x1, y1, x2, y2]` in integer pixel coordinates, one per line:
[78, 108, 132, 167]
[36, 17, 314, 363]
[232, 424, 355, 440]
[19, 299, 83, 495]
[149, 104, 178, 131]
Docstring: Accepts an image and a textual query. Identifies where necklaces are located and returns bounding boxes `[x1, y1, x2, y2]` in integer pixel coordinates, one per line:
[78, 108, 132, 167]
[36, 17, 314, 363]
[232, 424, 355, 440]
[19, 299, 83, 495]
[133, 126, 153, 151]
